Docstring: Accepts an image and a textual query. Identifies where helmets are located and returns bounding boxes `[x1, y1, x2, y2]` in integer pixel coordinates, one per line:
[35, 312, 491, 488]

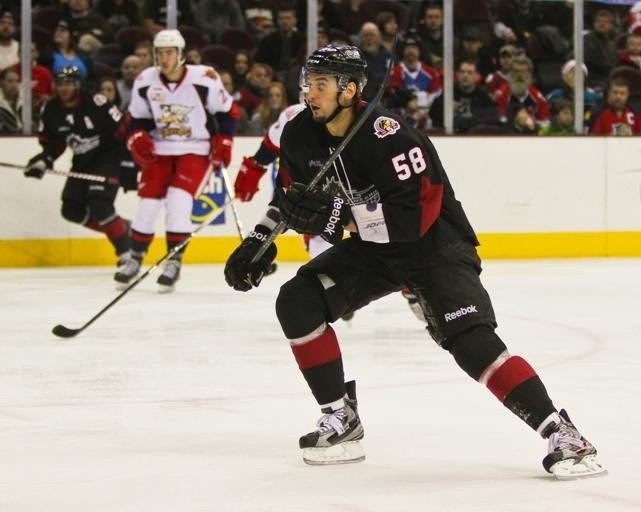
[54, 63, 81, 81]
[152, 29, 187, 73]
[305, 44, 368, 99]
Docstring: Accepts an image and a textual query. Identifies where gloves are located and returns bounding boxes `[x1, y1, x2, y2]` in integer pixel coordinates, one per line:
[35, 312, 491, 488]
[210, 135, 233, 172]
[276, 182, 351, 246]
[125, 128, 158, 168]
[233, 155, 268, 203]
[23, 154, 53, 180]
[224, 225, 278, 293]
[118, 166, 138, 194]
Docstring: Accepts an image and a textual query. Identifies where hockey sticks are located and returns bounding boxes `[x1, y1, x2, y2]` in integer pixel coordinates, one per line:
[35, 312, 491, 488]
[248, 30, 397, 267]
[220, 160, 276, 276]
[53, 198, 236, 336]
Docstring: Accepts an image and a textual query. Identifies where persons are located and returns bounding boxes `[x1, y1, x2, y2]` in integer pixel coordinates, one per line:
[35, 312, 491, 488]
[1, 1, 640, 137]
[235, 65, 429, 324]
[223, 39, 609, 477]
[113, 29, 241, 293]
[27, 52, 138, 270]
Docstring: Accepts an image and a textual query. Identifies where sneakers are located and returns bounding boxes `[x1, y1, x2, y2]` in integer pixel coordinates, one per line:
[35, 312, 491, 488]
[541, 422, 598, 472]
[113, 254, 142, 284]
[156, 257, 182, 287]
[299, 405, 365, 449]
